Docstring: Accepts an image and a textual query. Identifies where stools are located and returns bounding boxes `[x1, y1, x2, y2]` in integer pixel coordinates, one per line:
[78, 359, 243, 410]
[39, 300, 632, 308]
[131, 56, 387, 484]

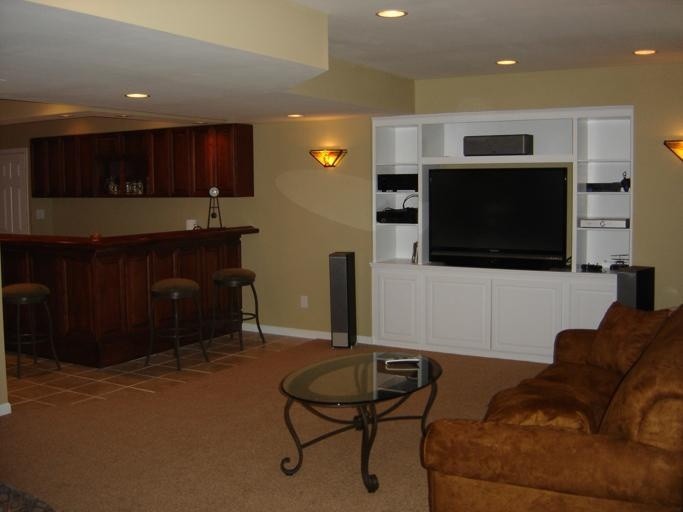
[142, 279, 211, 369]
[207, 268, 266, 351]
[2, 283, 62, 380]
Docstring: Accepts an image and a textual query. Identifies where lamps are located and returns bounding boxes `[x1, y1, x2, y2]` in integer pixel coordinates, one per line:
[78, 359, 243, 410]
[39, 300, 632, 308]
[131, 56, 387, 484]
[664, 140, 683, 163]
[309, 148, 347, 168]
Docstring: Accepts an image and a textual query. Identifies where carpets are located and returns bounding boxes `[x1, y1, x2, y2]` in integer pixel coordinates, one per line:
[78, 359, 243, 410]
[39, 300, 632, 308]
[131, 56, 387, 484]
[0, 339, 549, 512]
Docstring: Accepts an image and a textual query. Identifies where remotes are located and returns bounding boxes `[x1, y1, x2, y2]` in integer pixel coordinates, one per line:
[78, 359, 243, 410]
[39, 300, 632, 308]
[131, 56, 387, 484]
[385, 356, 420, 365]
[385, 365, 419, 371]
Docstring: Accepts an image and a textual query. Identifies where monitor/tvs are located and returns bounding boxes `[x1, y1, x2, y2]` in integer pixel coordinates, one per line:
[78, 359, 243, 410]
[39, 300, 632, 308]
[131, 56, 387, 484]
[428, 167, 568, 270]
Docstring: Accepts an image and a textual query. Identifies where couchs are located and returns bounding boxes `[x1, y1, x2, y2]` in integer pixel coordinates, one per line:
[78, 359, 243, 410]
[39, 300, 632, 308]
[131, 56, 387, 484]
[418, 302, 683, 512]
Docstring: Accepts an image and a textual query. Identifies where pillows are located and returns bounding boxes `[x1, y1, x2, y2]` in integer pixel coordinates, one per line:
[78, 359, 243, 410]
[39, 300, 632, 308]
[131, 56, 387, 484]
[587, 301, 670, 373]
[483, 384, 594, 433]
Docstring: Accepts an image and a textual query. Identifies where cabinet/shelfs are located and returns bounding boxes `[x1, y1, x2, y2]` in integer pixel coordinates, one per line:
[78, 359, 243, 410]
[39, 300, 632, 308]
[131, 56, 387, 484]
[371, 101, 638, 364]
[29, 122, 253, 200]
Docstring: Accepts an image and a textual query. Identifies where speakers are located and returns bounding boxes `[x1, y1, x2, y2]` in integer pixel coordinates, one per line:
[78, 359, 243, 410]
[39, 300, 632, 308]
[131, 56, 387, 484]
[618, 265, 655, 310]
[329, 251, 356, 349]
[464, 134, 533, 155]
